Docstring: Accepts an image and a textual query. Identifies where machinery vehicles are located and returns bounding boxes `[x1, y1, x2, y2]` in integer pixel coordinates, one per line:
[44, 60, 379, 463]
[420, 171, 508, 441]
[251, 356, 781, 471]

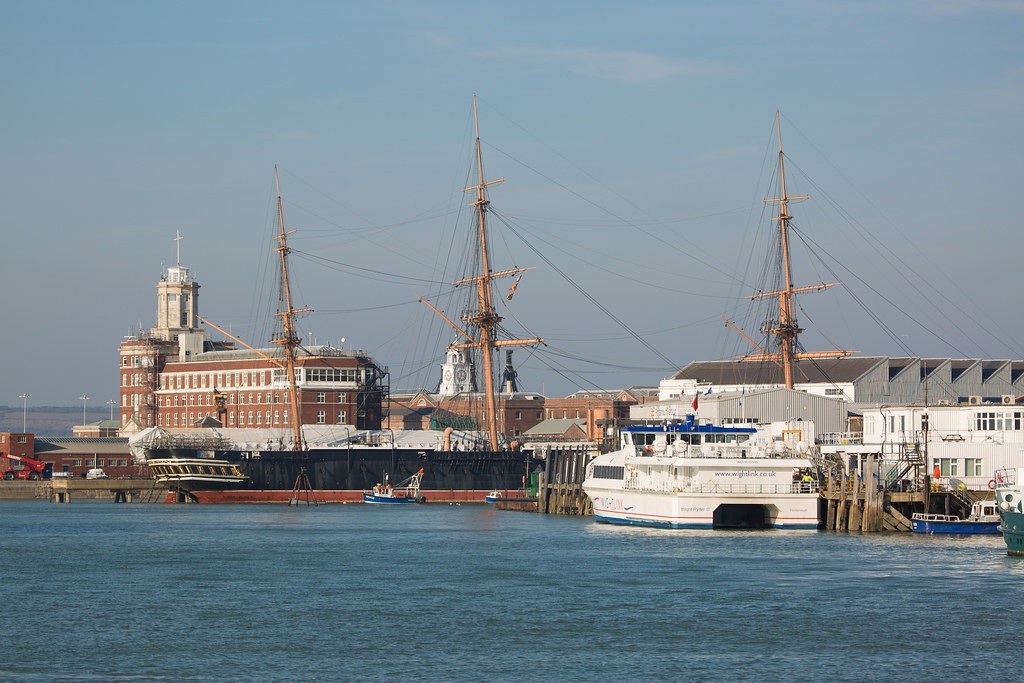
[0, 451, 45, 481]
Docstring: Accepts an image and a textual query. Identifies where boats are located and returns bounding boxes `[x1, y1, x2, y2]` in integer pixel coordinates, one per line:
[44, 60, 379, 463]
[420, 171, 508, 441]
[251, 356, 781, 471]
[994, 467, 1024, 556]
[363, 466, 426, 506]
[581, 415, 825, 530]
[910, 500, 1002, 534]
[485, 488, 502, 503]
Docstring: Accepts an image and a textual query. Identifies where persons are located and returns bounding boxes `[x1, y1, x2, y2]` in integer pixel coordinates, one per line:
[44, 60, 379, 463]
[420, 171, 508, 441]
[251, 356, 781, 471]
[801, 471, 815, 483]
[932, 464, 940, 492]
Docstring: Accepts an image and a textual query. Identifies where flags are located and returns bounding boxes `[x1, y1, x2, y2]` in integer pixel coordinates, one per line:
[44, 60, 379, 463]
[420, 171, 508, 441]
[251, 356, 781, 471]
[690, 393, 698, 411]
[506, 276, 521, 300]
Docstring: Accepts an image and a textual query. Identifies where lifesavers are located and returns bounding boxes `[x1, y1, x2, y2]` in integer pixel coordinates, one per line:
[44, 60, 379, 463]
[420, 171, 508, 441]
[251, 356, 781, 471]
[652, 439, 667, 452]
[672, 439, 687, 452]
[988, 479, 996, 488]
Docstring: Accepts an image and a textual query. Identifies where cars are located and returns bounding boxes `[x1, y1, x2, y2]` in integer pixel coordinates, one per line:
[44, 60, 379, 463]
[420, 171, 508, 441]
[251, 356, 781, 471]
[96, 474, 109, 479]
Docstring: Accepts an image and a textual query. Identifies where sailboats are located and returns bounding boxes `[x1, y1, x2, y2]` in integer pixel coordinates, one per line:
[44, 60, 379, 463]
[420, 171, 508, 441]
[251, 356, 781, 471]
[133, 92, 535, 506]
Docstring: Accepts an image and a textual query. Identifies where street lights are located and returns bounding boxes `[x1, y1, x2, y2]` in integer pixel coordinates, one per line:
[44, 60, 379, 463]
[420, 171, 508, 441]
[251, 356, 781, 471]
[79, 394, 90, 426]
[19, 393, 31, 433]
[106, 400, 117, 420]
